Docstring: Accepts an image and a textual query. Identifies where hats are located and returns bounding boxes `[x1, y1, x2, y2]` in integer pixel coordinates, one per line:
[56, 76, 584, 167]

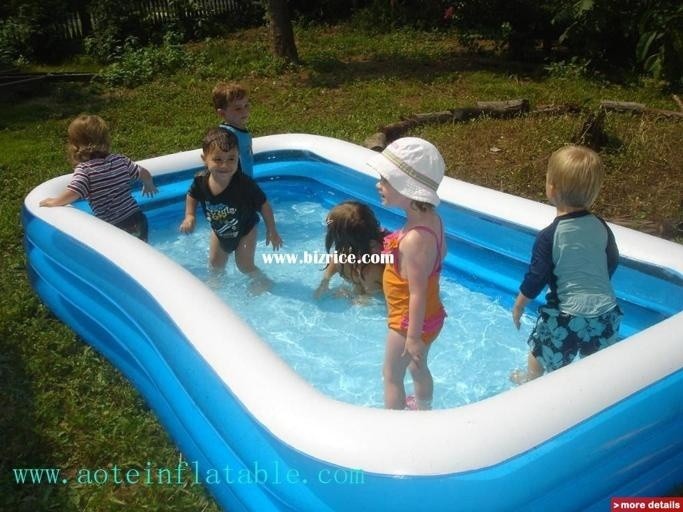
[365, 136, 446, 209]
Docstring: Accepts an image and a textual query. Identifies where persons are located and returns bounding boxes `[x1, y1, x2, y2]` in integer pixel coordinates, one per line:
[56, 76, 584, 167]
[39, 112, 162, 244]
[364, 135, 448, 411]
[506, 145, 620, 387]
[314, 201, 393, 306]
[179, 126, 286, 299]
[211, 81, 254, 178]
